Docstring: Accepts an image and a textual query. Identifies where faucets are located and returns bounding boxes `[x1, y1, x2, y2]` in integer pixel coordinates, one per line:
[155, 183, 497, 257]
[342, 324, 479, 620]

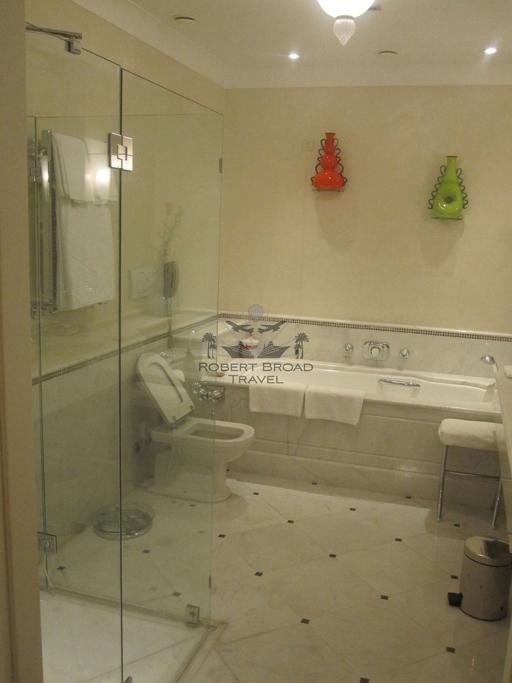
[481, 354, 500, 377]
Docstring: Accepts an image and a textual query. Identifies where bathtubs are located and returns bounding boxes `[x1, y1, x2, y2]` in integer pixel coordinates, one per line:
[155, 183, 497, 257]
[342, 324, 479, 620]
[150, 346, 503, 512]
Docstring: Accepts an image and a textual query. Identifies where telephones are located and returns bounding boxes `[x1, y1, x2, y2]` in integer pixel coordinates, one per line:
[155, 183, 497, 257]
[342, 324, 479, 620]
[164, 261, 179, 298]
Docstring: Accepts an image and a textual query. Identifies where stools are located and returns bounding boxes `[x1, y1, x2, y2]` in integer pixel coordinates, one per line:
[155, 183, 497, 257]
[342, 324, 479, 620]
[438, 418, 505, 528]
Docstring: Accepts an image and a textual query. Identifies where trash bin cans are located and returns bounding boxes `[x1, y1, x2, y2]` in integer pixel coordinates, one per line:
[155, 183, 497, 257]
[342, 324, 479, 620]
[448, 535, 512, 622]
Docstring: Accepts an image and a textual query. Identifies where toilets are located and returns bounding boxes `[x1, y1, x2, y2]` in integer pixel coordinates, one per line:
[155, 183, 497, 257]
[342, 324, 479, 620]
[134, 351, 255, 503]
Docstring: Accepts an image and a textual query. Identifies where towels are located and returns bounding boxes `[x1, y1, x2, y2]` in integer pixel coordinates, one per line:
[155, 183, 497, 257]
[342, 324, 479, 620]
[48, 133, 118, 312]
[247, 377, 366, 425]
